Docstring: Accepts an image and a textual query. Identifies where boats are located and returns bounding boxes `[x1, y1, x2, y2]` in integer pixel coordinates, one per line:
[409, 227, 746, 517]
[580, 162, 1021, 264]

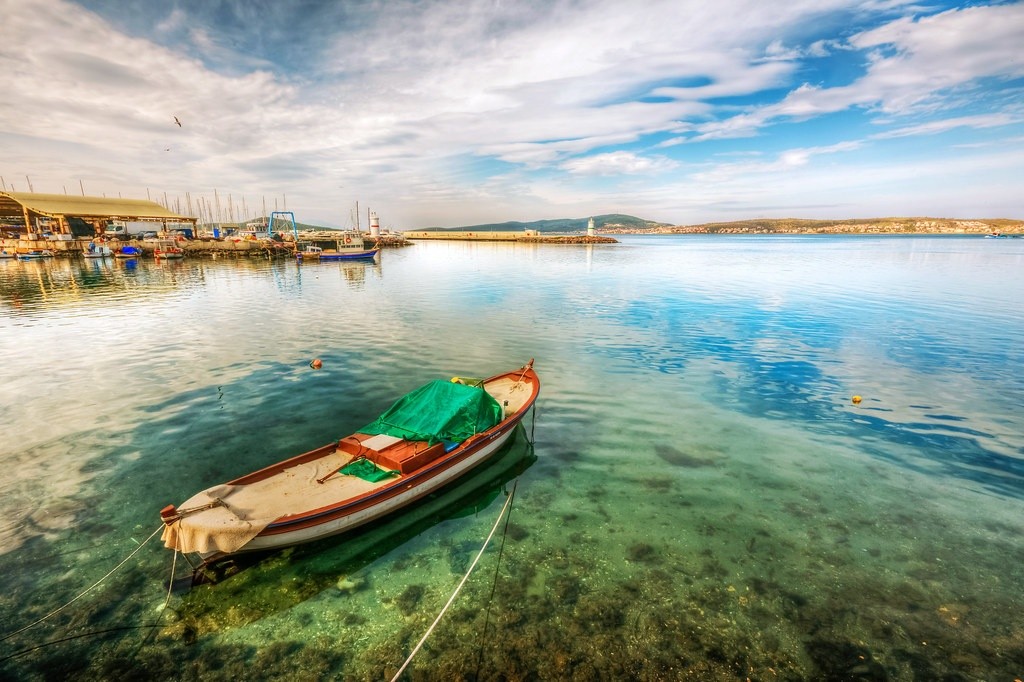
[0, 248, 57, 259]
[319, 201, 379, 259]
[152, 235, 183, 259]
[114, 246, 143, 258]
[160, 356, 539, 567]
[292, 244, 323, 259]
[83, 242, 113, 258]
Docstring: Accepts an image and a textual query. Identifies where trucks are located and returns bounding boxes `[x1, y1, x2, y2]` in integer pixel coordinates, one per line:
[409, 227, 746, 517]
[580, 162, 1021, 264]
[103, 221, 164, 241]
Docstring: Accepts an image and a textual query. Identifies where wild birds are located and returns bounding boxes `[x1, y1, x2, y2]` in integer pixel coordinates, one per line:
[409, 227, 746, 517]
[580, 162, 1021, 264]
[174, 116, 182, 127]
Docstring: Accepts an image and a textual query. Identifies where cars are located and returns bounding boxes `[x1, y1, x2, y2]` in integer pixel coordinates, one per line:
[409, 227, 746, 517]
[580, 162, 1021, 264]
[8, 230, 20, 239]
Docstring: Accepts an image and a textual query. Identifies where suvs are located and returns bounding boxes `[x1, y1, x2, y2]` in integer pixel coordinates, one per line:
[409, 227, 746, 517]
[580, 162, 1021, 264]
[130, 230, 157, 240]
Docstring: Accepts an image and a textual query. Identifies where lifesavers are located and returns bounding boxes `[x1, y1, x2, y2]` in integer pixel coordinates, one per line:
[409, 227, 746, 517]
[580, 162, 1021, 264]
[173, 248, 184, 253]
[100, 234, 109, 242]
[176, 236, 187, 248]
[346, 238, 351, 243]
[155, 249, 163, 254]
